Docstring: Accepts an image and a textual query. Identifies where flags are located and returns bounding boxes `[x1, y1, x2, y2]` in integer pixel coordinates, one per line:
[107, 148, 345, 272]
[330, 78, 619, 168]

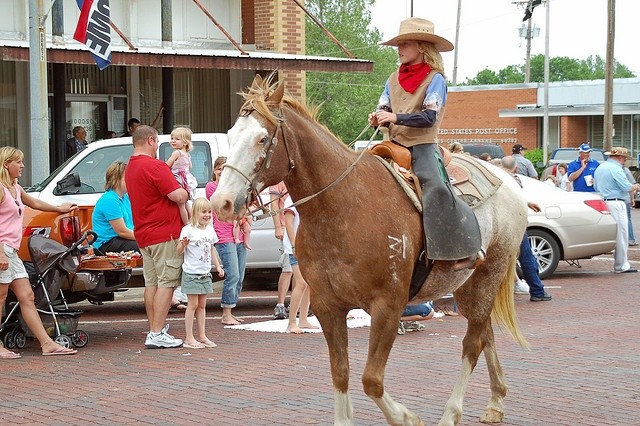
[73, 0, 112, 71]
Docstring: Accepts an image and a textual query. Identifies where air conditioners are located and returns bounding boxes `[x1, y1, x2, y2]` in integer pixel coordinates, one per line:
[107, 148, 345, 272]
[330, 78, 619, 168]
[355, 141, 384, 150]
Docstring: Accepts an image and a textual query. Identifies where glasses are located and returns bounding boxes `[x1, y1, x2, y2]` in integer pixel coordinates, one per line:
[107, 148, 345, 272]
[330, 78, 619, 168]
[116, 161, 122, 175]
[15, 199, 22, 215]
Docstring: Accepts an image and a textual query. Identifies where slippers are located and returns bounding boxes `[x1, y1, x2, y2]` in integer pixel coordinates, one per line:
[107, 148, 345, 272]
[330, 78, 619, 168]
[168, 302, 187, 312]
[204, 340, 218, 348]
[182, 342, 205, 348]
[0, 347, 22, 358]
[42, 347, 78, 355]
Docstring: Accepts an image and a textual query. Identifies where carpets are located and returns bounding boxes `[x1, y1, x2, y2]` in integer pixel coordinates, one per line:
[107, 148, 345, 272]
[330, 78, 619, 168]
[224, 308, 446, 333]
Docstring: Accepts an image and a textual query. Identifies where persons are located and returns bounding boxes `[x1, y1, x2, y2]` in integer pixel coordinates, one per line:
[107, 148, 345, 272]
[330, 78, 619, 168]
[91, 162, 187, 310]
[124, 125, 193, 348]
[67, 126, 88, 159]
[511, 144, 538, 179]
[623, 167, 639, 246]
[479, 152, 491, 162]
[177, 196, 224, 349]
[367, 16, 478, 272]
[232, 208, 251, 250]
[269, 181, 315, 320]
[103, 131, 116, 140]
[120, 103, 163, 137]
[593, 147, 640, 274]
[0, 146, 78, 359]
[567, 143, 600, 192]
[502, 155, 518, 173]
[283, 195, 320, 334]
[448, 142, 464, 155]
[398, 303, 434, 335]
[558, 163, 571, 192]
[205, 155, 252, 325]
[165, 127, 198, 225]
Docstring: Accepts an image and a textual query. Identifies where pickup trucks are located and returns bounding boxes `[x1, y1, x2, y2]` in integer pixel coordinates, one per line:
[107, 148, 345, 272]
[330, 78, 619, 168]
[17, 132, 281, 305]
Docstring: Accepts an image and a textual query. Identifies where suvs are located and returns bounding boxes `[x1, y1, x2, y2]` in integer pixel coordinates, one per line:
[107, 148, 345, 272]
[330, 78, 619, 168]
[456, 142, 504, 159]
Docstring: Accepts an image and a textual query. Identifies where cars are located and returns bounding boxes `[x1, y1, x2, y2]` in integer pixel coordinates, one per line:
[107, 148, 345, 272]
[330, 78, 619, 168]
[513, 173, 617, 280]
[536, 147, 609, 169]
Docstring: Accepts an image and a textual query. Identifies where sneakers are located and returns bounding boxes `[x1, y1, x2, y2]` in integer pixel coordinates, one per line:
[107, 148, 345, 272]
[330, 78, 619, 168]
[286, 304, 299, 316]
[530, 292, 550, 301]
[403, 319, 424, 331]
[630, 242, 639, 245]
[145, 332, 183, 348]
[394, 320, 405, 335]
[273, 305, 287, 319]
[622, 268, 637, 272]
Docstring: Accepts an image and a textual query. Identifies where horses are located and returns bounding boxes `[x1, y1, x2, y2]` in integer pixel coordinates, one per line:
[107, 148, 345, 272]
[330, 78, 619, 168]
[207, 67, 530, 426]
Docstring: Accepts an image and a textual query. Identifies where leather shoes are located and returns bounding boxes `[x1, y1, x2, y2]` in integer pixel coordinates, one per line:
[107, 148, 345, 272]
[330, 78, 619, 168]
[453, 257, 474, 270]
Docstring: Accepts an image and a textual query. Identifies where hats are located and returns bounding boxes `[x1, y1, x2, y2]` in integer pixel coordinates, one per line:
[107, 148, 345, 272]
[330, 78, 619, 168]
[604, 146, 632, 159]
[377, 17, 454, 51]
[512, 144, 527, 151]
[578, 142, 592, 152]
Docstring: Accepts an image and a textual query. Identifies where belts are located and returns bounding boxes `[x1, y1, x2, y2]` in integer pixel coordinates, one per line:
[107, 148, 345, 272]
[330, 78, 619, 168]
[604, 198, 624, 201]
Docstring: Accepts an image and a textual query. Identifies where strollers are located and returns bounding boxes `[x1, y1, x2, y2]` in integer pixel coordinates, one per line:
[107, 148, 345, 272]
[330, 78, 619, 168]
[0, 229, 99, 349]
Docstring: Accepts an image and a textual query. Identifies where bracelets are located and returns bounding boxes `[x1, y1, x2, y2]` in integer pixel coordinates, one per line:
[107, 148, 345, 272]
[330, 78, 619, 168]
[291, 245, 295, 249]
